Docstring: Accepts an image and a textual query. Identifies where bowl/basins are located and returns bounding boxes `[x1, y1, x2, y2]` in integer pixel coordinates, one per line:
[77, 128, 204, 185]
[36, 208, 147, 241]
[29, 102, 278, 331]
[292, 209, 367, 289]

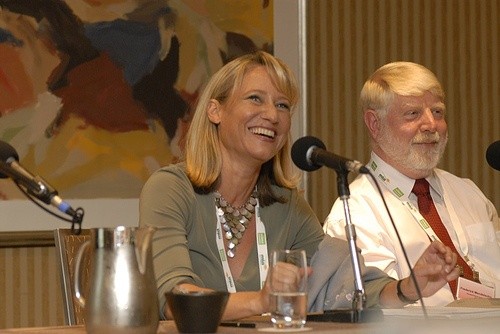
[163, 291, 230, 334]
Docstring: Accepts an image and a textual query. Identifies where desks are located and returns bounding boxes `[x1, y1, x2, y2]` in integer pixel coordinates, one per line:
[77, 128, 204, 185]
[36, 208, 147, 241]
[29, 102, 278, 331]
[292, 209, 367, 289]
[1, 300, 499, 333]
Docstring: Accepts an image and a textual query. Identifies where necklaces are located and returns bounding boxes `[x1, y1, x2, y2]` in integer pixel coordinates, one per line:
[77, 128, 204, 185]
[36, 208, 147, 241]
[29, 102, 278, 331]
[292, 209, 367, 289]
[211, 185, 259, 258]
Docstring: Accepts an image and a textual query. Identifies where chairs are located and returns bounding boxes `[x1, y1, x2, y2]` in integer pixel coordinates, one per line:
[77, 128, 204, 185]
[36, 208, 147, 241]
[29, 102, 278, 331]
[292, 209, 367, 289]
[55, 224, 95, 325]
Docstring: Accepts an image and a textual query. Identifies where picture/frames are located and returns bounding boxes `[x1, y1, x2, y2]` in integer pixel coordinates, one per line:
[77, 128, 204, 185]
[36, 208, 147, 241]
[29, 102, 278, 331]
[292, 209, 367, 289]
[0, 0, 308, 245]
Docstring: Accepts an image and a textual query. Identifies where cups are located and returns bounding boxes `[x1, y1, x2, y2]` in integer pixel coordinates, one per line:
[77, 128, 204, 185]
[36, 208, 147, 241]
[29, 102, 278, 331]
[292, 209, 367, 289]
[268, 249, 307, 330]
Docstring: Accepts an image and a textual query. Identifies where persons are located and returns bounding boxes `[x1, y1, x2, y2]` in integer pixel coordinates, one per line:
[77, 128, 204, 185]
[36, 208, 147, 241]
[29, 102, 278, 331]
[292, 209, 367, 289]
[139, 50, 460, 320]
[323, 62, 500, 306]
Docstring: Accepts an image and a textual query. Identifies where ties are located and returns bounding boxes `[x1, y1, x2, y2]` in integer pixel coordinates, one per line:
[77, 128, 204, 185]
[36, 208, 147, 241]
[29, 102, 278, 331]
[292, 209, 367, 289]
[411, 180, 481, 300]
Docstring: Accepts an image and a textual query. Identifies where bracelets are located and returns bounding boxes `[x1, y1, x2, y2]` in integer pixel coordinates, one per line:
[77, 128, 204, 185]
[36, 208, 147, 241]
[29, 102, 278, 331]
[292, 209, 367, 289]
[396, 279, 419, 304]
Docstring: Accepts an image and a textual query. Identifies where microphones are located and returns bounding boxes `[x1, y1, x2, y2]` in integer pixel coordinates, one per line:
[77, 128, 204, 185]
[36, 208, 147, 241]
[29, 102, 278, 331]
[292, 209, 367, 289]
[291, 137, 363, 174]
[486, 140, 500, 170]
[0, 141, 78, 217]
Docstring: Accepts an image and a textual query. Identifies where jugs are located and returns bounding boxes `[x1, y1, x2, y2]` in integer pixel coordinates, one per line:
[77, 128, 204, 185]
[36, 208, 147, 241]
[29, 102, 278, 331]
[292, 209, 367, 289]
[72, 226, 161, 334]
